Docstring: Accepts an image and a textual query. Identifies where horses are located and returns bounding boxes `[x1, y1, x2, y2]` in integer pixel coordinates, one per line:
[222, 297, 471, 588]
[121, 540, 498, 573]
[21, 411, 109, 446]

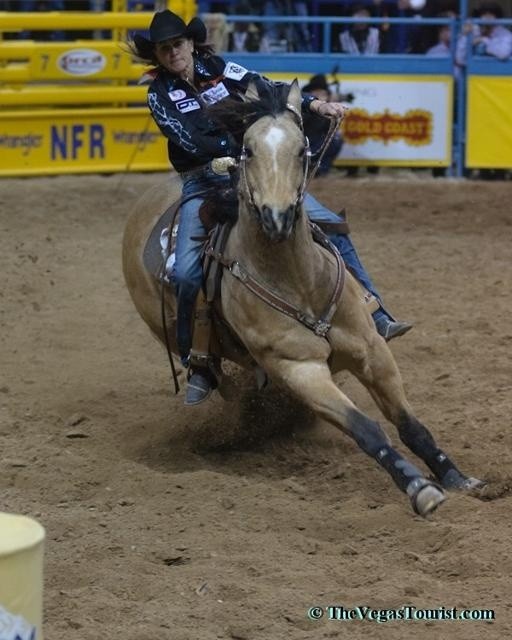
[118, 73, 488, 519]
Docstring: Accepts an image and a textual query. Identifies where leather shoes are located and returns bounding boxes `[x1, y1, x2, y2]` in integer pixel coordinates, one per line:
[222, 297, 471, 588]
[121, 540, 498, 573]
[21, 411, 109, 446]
[183, 368, 213, 405]
[378, 319, 413, 343]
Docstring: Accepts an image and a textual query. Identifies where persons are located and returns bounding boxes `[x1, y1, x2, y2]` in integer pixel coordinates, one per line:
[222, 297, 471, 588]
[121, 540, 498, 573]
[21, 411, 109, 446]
[119, 8, 414, 405]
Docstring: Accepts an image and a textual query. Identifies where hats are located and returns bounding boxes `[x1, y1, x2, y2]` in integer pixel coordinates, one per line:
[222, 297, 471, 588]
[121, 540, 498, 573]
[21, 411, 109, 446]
[301, 73, 332, 94]
[133, 10, 207, 59]
